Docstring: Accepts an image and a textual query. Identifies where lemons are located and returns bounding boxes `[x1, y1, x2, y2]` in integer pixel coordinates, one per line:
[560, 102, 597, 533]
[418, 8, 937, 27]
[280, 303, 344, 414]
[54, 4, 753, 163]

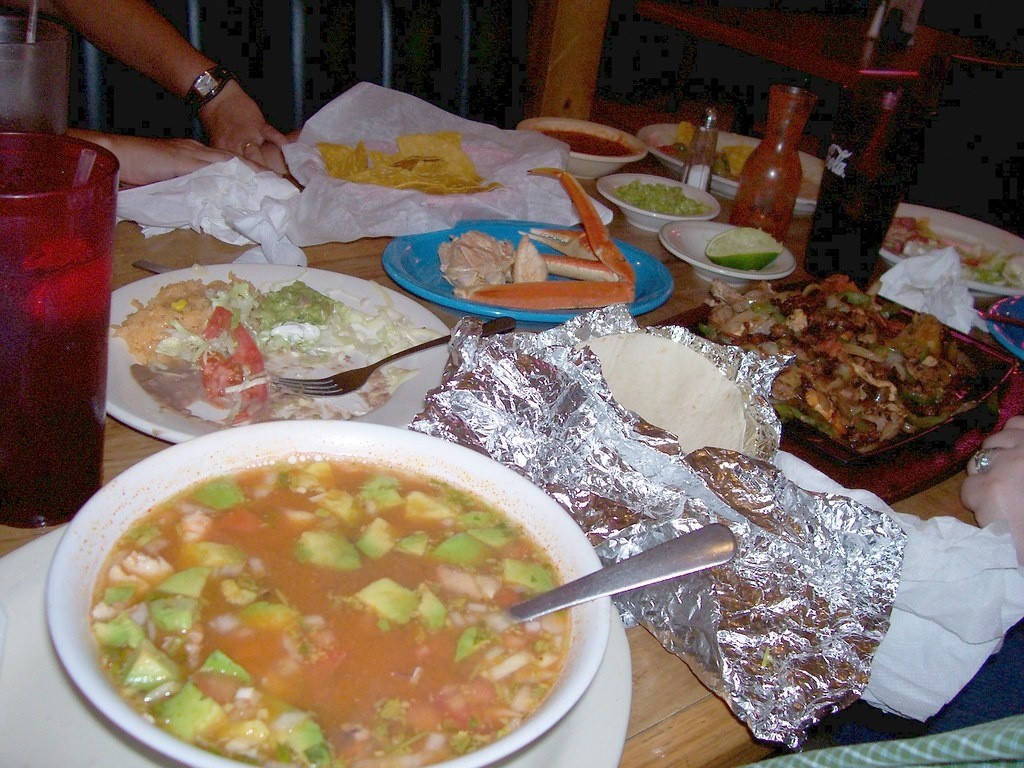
[707, 228, 783, 269]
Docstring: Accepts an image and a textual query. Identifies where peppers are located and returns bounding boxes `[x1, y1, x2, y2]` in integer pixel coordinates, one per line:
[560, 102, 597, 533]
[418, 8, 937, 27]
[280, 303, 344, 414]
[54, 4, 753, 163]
[700, 291, 949, 432]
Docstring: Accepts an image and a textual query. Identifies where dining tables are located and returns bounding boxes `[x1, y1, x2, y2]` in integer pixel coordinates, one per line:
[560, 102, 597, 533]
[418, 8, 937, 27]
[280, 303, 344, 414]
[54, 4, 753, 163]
[0, 167, 1024, 768]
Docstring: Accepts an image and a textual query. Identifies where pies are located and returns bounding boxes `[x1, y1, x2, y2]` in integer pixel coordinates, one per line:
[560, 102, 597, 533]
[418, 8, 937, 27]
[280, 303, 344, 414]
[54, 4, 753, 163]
[568, 332, 744, 458]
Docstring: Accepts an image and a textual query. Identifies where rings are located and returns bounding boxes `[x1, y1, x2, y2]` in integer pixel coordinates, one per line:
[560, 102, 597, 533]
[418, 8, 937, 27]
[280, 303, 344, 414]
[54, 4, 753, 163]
[974, 445, 1002, 473]
[244, 142, 257, 146]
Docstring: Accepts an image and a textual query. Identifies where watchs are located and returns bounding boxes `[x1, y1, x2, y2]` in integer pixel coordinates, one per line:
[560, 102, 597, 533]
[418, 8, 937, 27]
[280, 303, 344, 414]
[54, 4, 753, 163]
[183, 65, 239, 112]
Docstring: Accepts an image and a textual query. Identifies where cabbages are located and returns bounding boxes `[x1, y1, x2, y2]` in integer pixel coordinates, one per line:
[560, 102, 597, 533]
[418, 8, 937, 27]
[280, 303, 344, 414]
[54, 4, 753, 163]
[156, 279, 443, 395]
[961, 251, 1024, 290]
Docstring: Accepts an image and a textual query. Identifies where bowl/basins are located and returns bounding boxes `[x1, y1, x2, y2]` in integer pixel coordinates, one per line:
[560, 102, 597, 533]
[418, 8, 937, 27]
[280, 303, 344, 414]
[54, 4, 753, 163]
[515, 117, 650, 179]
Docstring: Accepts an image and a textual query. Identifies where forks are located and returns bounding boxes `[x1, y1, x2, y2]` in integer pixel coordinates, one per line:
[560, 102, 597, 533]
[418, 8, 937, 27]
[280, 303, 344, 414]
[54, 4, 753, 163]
[275, 315, 515, 395]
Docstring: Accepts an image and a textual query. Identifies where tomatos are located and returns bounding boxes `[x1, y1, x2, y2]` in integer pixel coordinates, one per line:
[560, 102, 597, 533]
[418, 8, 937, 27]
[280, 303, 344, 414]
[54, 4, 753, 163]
[204, 304, 266, 424]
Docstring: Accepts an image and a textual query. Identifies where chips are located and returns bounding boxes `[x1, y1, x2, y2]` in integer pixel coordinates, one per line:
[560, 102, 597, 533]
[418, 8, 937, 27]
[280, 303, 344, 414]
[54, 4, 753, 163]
[313, 131, 502, 195]
[676, 123, 756, 177]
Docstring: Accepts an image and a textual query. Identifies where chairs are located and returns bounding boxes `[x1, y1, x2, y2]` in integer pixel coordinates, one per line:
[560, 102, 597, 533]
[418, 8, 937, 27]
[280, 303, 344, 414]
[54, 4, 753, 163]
[526, 0, 972, 184]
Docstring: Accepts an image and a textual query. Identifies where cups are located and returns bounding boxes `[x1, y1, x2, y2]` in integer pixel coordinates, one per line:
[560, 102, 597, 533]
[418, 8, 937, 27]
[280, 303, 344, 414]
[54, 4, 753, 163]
[0, 127, 123, 529]
[802, 81, 935, 286]
[0, 10, 73, 135]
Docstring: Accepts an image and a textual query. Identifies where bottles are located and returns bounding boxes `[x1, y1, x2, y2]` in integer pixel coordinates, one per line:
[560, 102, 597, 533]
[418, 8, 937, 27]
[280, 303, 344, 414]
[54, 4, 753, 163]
[677, 107, 720, 196]
[727, 82, 818, 244]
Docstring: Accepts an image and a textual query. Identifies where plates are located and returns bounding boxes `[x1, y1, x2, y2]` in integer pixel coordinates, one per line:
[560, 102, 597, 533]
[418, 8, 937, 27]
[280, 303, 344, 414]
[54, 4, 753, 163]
[881, 201, 1024, 301]
[595, 173, 721, 232]
[0, 511, 633, 768]
[636, 121, 826, 216]
[46, 418, 609, 767]
[984, 294, 1023, 365]
[106, 263, 451, 443]
[658, 220, 797, 289]
[382, 215, 673, 325]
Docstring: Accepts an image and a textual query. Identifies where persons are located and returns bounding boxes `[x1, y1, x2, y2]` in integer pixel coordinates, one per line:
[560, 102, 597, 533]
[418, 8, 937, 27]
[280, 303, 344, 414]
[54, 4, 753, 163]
[960, 414, 1024, 567]
[0, 0, 291, 187]
